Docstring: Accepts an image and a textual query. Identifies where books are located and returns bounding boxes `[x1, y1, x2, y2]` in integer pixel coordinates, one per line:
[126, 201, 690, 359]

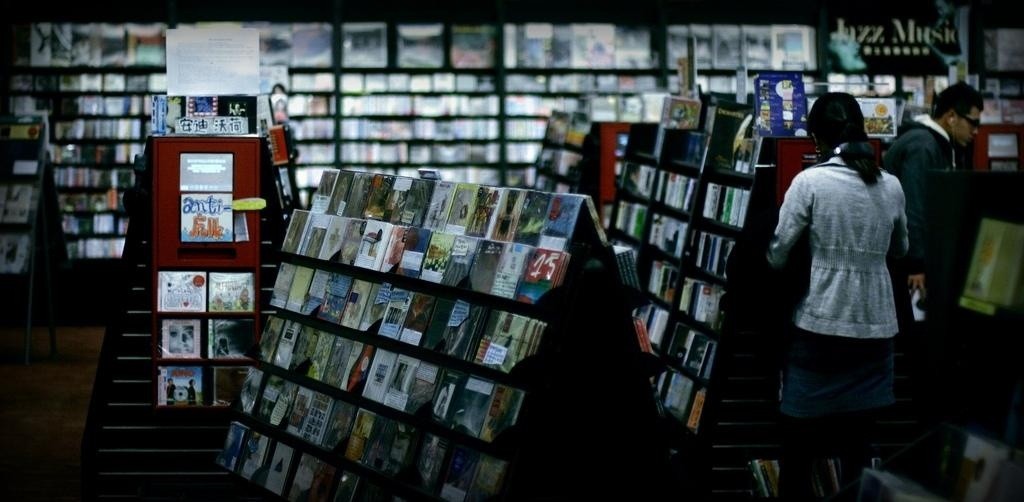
[823, 70, 1022, 173]
[963, 218, 1023, 310]
[736, 416, 1024, 501]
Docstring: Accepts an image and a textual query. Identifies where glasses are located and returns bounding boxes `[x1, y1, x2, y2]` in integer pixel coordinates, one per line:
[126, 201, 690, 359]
[957, 111, 980, 127]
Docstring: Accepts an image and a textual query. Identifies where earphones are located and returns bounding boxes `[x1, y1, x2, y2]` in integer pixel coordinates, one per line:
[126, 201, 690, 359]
[950, 117, 954, 123]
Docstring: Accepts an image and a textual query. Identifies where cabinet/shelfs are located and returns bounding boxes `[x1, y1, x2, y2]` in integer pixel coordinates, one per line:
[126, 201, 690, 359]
[0, 0, 1024, 502]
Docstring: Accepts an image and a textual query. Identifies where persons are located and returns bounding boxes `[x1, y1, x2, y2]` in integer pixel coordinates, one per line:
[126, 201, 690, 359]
[878, 80, 1008, 483]
[764, 90, 909, 501]
